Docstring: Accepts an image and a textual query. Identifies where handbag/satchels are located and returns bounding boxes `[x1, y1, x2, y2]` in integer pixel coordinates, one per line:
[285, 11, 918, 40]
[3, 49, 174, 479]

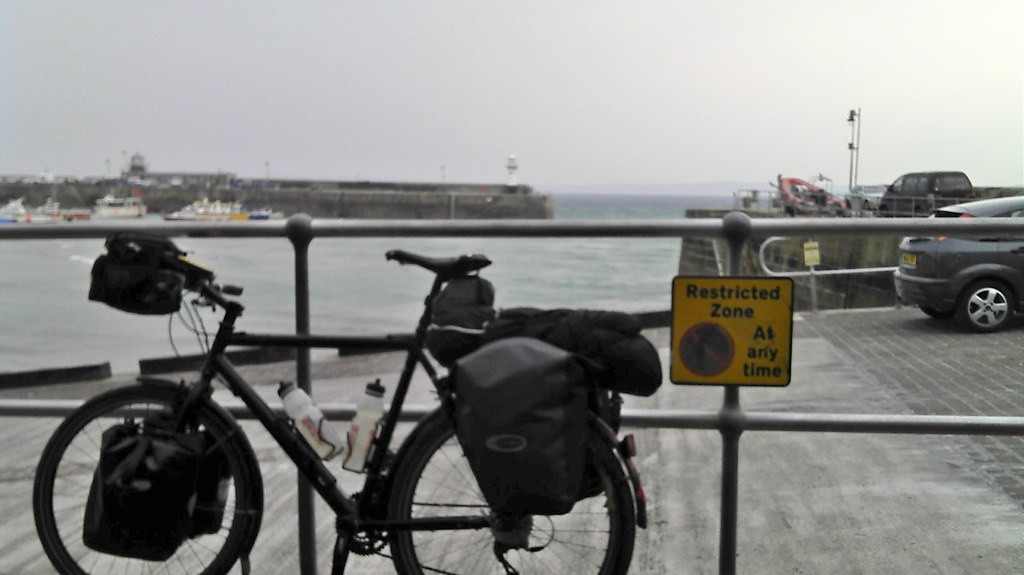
[83, 424, 203, 560]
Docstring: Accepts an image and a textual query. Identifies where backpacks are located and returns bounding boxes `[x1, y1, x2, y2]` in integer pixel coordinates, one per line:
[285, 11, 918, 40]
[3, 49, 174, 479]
[436, 336, 608, 575]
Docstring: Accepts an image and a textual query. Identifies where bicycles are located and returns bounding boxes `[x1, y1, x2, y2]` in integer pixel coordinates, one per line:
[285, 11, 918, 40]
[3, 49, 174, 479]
[33, 233, 663, 575]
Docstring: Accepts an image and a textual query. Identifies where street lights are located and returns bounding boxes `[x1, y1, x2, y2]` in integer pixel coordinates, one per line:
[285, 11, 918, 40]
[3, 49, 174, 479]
[848, 110, 861, 186]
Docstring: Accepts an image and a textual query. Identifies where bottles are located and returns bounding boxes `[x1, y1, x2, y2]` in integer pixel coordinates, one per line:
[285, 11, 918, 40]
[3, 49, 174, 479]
[277, 379, 346, 460]
[342, 379, 386, 473]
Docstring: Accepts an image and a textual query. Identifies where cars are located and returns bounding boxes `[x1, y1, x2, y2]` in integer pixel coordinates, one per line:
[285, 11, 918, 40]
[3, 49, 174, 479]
[894, 196, 1024, 333]
[845, 184, 888, 210]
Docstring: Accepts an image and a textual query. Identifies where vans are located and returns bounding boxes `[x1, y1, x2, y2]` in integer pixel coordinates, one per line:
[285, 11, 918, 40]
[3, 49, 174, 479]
[880, 171, 976, 218]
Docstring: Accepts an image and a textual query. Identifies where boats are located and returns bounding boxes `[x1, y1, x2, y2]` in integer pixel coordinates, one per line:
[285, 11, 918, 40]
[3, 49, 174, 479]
[94, 197, 147, 219]
[163, 198, 272, 221]
[37, 198, 91, 221]
[0, 198, 63, 223]
